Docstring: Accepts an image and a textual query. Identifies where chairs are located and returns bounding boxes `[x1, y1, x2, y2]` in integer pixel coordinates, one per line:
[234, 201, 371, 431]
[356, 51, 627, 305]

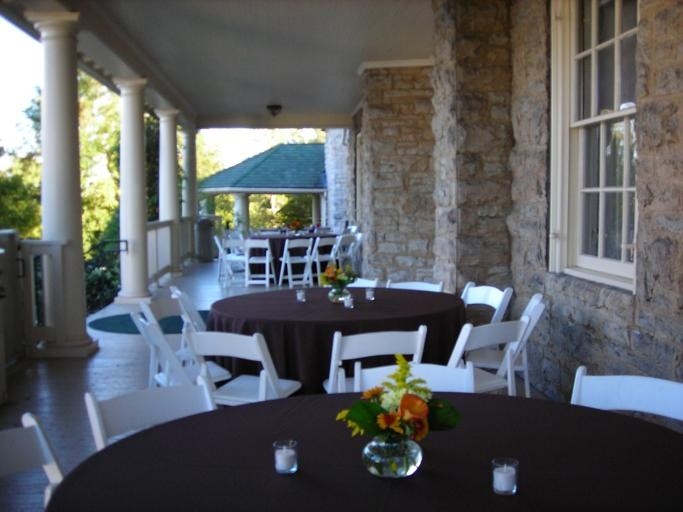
[85, 376, 218, 450]
[0, 413, 65, 512]
[213, 221, 365, 288]
[570, 366, 683, 427]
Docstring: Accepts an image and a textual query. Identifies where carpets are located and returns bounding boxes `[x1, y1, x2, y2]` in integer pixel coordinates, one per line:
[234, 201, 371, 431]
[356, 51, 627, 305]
[88, 310, 209, 335]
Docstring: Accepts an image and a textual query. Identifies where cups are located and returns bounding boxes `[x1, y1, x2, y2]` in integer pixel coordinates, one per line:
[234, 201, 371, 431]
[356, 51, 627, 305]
[295, 289, 307, 304]
[272, 439, 299, 474]
[342, 295, 355, 310]
[238, 224, 331, 236]
[365, 287, 375, 301]
[490, 456, 520, 496]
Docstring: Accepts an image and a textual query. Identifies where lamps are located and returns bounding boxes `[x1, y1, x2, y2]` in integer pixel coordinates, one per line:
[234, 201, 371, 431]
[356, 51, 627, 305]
[267, 104, 282, 117]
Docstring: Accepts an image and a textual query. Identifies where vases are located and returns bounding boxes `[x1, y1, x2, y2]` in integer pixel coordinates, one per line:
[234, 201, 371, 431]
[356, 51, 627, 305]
[361, 436, 423, 478]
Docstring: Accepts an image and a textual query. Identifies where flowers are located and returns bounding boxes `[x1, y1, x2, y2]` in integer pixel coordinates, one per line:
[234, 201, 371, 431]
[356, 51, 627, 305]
[335, 355, 463, 475]
